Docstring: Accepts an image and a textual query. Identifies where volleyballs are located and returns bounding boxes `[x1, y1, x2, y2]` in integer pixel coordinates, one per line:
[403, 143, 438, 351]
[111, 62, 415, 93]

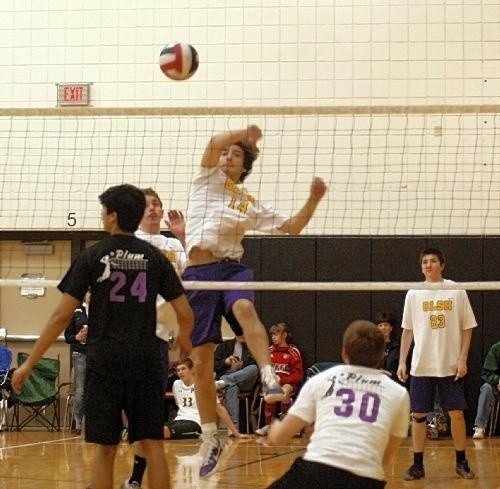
[158, 42, 198, 80]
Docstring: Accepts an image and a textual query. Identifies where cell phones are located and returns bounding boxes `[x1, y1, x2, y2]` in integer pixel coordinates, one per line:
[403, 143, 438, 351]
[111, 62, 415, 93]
[233, 355, 240, 361]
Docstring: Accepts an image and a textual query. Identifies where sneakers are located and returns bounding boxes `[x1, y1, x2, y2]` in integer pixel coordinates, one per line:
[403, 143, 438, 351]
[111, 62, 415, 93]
[260, 363, 286, 404]
[199, 438, 225, 479]
[455, 459, 476, 479]
[472, 427, 485, 439]
[255, 424, 271, 435]
[404, 464, 426, 479]
[437, 420, 449, 435]
[426, 424, 438, 439]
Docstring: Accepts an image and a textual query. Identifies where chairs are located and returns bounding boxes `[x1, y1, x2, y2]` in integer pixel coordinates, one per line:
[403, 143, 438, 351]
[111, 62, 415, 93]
[237, 362, 344, 437]
[0, 346, 12, 435]
[13, 350, 62, 433]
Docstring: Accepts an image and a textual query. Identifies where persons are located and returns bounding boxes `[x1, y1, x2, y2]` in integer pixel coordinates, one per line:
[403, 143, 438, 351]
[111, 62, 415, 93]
[255, 323, 303, 436]
[64, 285, 96, 434]
[396, 248, 479, 480]
[375, 312, 408, 389]
[163, 358, 251, 440]
[473, 342, 500, 439]
[119, 187, 184, 489]
[180, 125, 327, 479]
[213, 334, 260, 436]
[268, 320, 411, 488]
[11, 184, 194, 489]
